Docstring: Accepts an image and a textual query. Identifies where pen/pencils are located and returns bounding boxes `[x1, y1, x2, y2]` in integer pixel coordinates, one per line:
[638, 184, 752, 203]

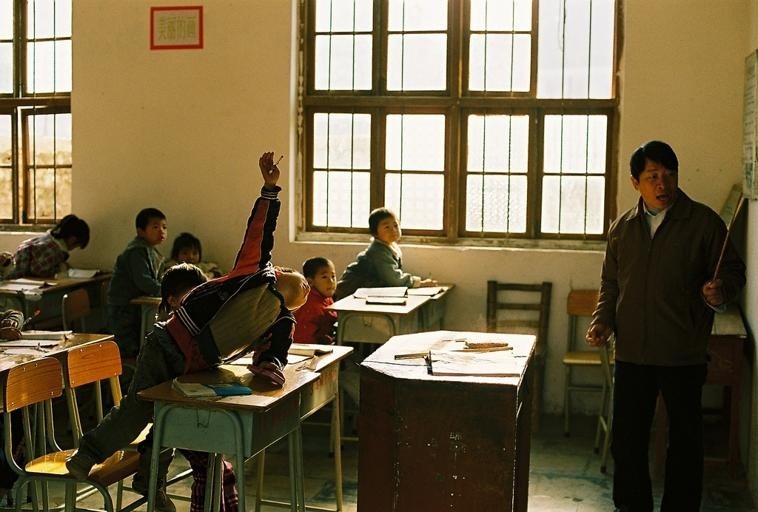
[17, 310, 41, 329]
[269, 156, 284, 175]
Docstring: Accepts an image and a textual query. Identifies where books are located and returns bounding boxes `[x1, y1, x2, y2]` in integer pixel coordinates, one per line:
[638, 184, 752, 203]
[53, 266, 113, 277]
[350, 279, 409, 299]
[404, 282, 449, 297]
[171, 378, 254, 400]
[0, 276, 59, 294]
[428, 346, 522, 377]
[229, 337, 257, 365]
[285, 341, 333, 364]
[0, 324, 75, 358]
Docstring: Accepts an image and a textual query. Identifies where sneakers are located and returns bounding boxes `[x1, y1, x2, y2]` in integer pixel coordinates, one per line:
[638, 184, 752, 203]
[132, 472, 177, 512]
[66, 448, 92, 482]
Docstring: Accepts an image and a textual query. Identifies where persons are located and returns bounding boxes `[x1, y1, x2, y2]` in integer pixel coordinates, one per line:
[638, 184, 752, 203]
[11, 214, 91, 277]
[585, 139, 751, 512]
[0, 309, 24, 490]
[164, 228, 222, 277]
[332, 207, 439, 304]
[104, 207, 168, 358]
[0, 250, 17, 281]
[293, 256, 363, 407]
[63, 149, 311, 511]
[156, 260, 243, 512]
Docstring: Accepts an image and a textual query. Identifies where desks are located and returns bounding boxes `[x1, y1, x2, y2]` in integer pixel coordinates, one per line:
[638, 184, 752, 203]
[656, 303, 748, 481]
